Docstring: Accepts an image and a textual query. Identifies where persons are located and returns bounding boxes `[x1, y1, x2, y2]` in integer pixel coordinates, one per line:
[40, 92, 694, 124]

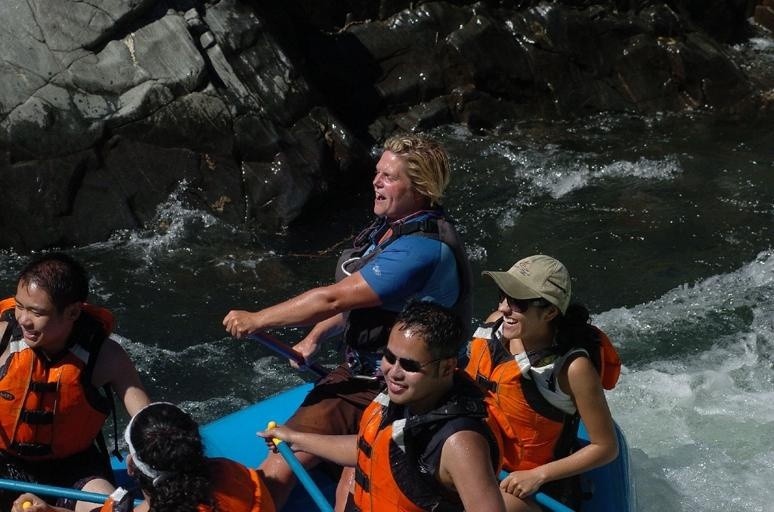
[0, 252, 152, 512]
[221, 131, 469, 512]
[464, 254, 620, 512]
[255, 300, 507, 512]
[10, 402, 275, 512]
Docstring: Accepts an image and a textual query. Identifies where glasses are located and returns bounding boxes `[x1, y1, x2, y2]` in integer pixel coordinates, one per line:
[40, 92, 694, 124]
[496, 287, 551, 313]
[383, 349, 450, 371]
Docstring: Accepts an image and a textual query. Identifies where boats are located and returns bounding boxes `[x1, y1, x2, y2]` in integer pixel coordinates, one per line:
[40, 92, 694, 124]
[109, 378, 632, 512]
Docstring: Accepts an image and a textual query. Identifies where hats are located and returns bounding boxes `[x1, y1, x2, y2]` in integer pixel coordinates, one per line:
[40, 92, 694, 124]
[481, 254, 572, 316]
